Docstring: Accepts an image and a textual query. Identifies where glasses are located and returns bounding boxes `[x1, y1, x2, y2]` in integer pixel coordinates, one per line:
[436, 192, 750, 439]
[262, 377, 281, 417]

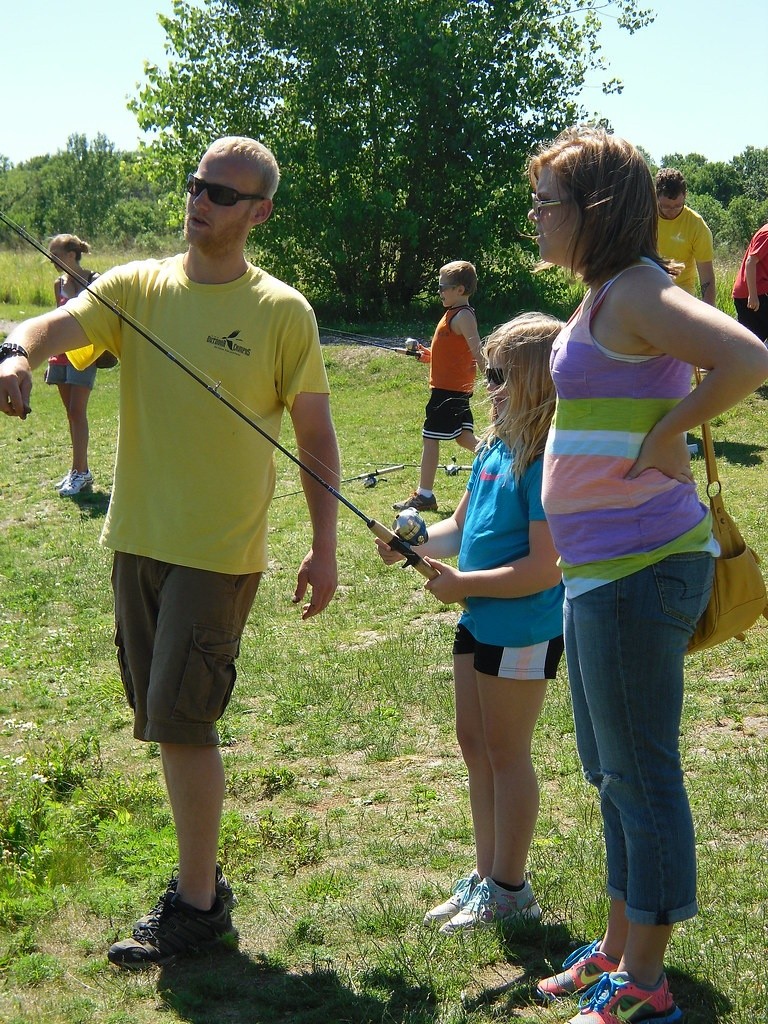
[439, 284, 457, 293]
[185, 172, 266, 208]
[486, 367, 505, 386]
[530, 192, 570, 218]
[658, 200, 683, 212]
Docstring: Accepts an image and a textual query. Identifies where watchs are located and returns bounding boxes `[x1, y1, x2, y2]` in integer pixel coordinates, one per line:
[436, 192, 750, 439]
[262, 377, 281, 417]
[0, 343, 28, 360]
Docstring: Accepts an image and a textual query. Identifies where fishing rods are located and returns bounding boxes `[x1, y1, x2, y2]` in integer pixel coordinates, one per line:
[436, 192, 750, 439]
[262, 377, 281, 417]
[318, 330, 426, 359]
[0, 214, 470, 614]
[366, 456, 473, 477]
[272, 465, 404, 501]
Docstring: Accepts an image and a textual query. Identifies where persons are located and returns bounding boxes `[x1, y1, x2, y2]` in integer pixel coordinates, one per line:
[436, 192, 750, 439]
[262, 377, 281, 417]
[44, 234, 102, 496]
[654, 168, 716, 307]
[375, 311, 564, 936]
[733, 222, 768, 342]
[393, 261, 488, 511]
[0, 137, 340, 970]
[528, 134, 768, 1024]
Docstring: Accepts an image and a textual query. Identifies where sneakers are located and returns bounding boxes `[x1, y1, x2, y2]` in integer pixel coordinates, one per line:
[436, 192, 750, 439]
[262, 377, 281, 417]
[54, 469, 95, 496]
[440, 879, 542, 934]
[391, 490, 438, 511]
[565, 969, 680, 1024]
[134, 864, 239, 934]
[107, 892, 239, 970]
[538, 938, 620, 1000]
[422, 866, 481, 927]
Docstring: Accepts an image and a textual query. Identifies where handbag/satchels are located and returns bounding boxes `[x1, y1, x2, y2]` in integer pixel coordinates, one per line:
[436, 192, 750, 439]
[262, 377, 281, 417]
[88, 271, 118, 370]
[682, 494, 768, 656]
